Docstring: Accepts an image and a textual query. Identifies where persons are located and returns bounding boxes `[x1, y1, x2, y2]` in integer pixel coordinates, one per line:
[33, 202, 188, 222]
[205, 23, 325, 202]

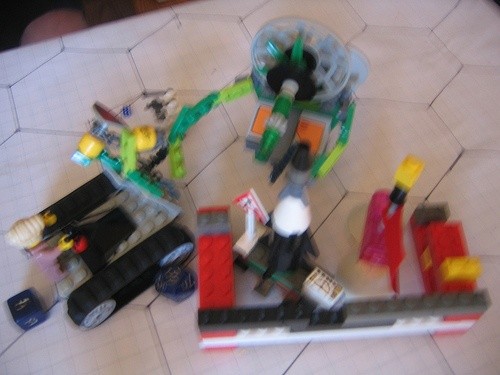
[0, 0, 88, 52]
